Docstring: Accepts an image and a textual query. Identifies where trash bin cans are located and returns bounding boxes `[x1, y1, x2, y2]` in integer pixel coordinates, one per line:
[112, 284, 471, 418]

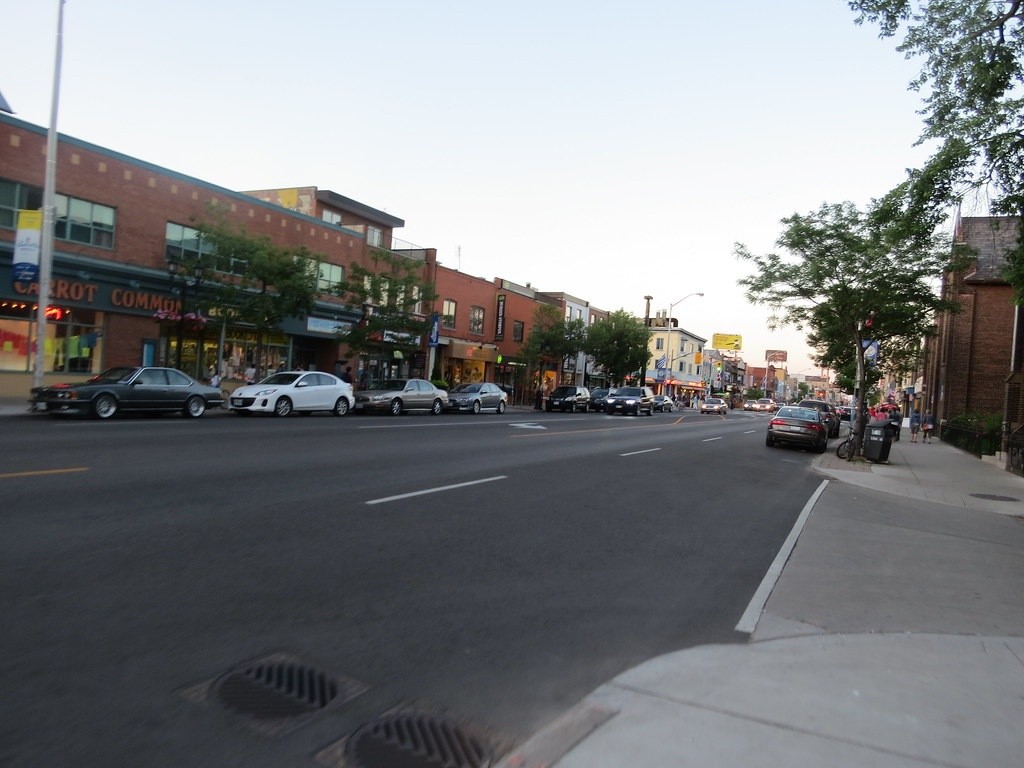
[863, 419, 895, 463]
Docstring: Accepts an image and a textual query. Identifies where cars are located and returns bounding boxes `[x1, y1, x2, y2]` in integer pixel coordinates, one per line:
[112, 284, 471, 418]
[228, 371, 355, 417]
[353, 379, 450, 416]
[448, 383, 508, 414]
[743, 398, 786, 413]
[27, 366, 225, 420]
[766, 405, 829, 454]
[655, 395, 674, 413]
[701, 398, 728, 414]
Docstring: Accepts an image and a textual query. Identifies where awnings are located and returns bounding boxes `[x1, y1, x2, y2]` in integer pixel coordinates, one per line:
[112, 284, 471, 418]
[447, 337, 499, 363]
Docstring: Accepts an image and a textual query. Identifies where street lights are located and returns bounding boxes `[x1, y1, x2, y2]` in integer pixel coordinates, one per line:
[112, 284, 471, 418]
[167, 254, 205, 379]
[663, 292, 704, 395]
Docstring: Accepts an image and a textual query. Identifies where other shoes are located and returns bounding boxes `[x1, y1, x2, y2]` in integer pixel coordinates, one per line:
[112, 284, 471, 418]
[929, 441, 931, 444]
[910, 440, 913, 442]
[914, 441, 916, 443]
[923, 440, 925, 443]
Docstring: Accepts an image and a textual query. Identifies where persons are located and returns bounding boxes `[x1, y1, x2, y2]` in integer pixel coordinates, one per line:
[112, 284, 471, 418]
[244, 363, 304, 385]
[922, 410, 936, 444]
[671, 392, 706, 410]
[340, 366, 352, 383]
[909, 409, 921, 442]
[732, 396, 736, 408]
[868, 405, 903, 442]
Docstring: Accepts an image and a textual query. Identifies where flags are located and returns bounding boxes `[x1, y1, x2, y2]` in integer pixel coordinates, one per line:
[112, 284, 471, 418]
[695, 352, 702, 364]
[887, 389, 896, 400]
[657, 354, 666, 368]
[862, 341, 878, 367]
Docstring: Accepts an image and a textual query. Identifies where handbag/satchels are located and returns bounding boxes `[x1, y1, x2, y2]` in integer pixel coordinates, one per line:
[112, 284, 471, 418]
[923, 423, 928, 431]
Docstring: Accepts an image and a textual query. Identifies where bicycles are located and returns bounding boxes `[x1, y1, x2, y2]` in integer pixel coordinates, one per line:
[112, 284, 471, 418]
[836, 425, 858, 462]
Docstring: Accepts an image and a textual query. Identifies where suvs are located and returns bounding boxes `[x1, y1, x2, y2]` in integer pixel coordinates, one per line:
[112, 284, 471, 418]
[546, 384, 590, 413]
[590, 386, 655, 416]
[797, 400, 857, 438]
